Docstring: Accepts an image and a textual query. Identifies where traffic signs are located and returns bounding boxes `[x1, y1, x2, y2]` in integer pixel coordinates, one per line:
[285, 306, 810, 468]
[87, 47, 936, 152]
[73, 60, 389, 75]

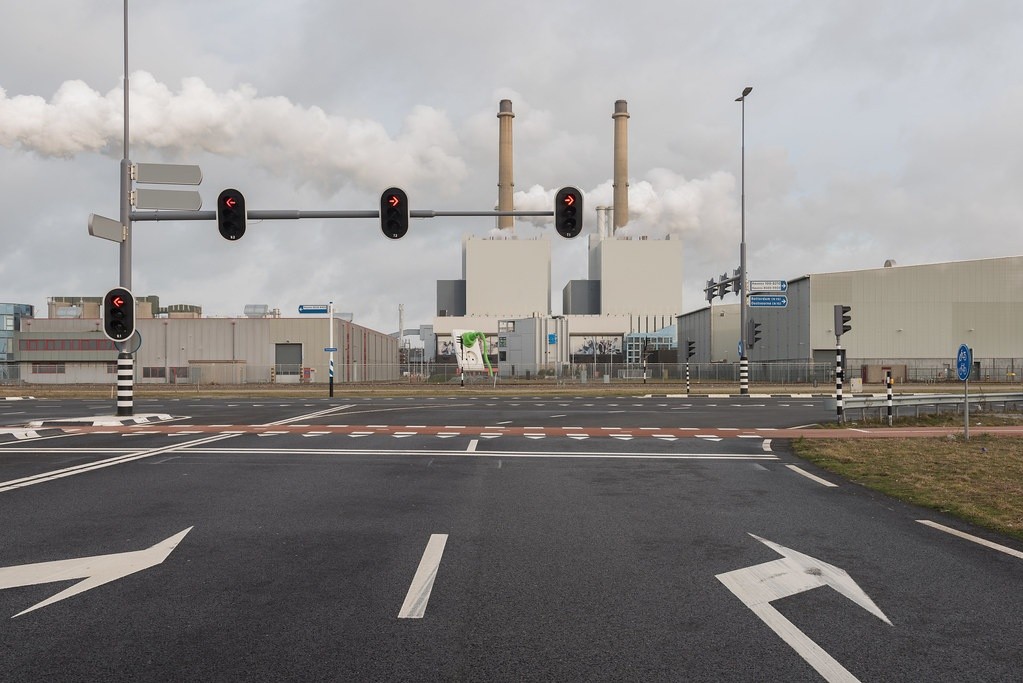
[297, 304, 329, 314]
[749, 279, 788, 292]
[748, 295, 788, 308]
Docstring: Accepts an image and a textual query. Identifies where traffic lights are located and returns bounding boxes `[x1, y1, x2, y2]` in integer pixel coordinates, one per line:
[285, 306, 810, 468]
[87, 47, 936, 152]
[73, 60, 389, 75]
[644, 334, 651, 348]
[378, 185, 410, 241]
[834, 304, 851, 335]
[684, 337, 696, 359]
[217, 188, 247, 242]
[102, 288, 136, 342]
[748, 318, 763, 347]
[733, 266, 748, 296]
[708, 278, 718, 304]
[553, 185, 584, 240]
[718, 272, 732, 300]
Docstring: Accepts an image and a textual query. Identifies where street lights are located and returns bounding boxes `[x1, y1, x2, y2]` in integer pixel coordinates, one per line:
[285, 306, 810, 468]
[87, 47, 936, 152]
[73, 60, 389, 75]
[734, 85, 753, 394]
